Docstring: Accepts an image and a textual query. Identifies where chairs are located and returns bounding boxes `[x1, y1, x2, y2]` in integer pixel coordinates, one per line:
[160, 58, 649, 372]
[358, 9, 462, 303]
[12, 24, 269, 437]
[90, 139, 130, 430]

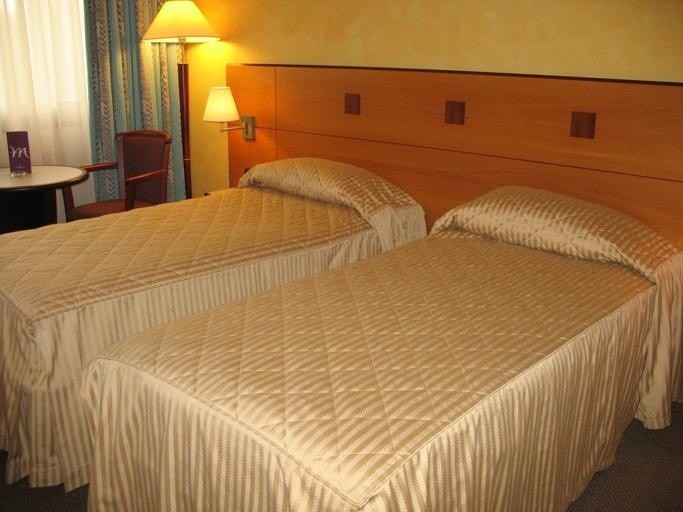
[62, 130, 171, 222]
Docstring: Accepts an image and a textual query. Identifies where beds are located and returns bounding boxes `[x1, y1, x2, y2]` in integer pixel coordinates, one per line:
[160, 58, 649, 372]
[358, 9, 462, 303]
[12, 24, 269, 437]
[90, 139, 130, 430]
[0, 186, 381, 493]
[80, 229, 657, 512]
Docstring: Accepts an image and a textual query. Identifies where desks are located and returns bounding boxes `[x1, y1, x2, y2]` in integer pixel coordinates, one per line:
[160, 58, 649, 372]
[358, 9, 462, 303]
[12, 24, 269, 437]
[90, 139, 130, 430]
[0, 166, 89, 233]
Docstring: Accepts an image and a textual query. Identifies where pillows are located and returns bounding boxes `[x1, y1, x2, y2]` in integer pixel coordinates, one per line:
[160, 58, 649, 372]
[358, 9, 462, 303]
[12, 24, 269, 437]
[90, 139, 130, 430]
[430, 185, 683, 285]
[238, 157, 425, 234]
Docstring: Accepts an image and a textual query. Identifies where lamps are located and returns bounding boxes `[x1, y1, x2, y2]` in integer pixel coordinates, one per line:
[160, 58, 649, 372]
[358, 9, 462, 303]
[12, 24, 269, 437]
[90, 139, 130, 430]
[203, 87, 254, 139]
[142, 0, 222, 199]
